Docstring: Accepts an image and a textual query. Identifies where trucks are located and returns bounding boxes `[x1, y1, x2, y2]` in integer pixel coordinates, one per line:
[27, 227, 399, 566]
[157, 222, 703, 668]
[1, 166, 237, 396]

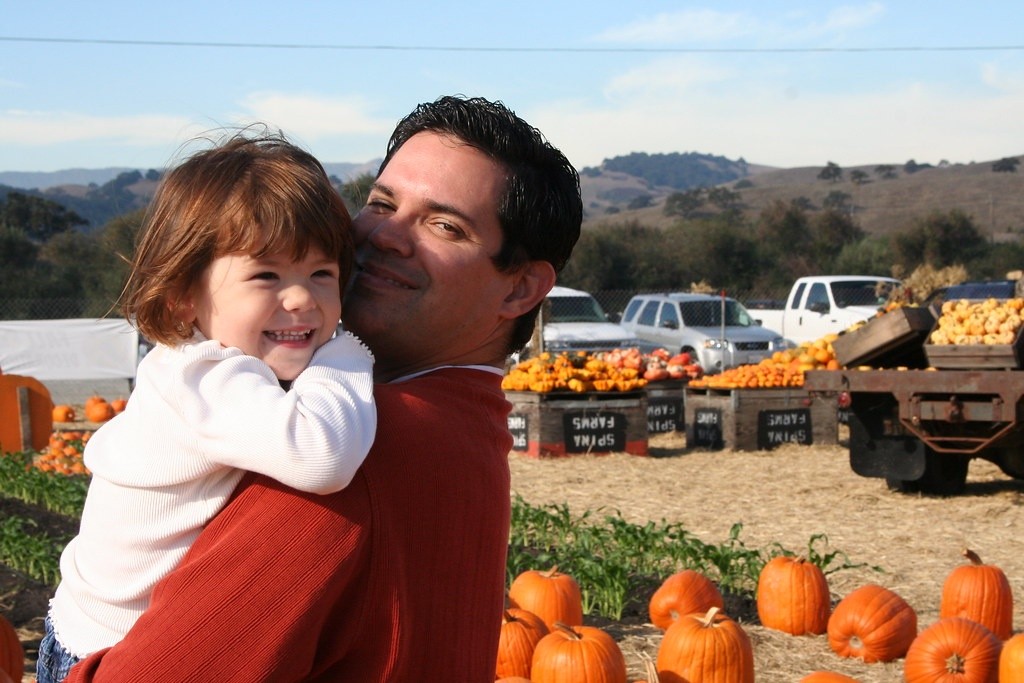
[802, 276, 1024, 498]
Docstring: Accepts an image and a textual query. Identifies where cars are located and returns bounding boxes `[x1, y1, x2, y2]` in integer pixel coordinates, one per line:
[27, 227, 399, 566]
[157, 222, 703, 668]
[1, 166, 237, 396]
[920, 280, 1016, 306]
[622, 291, 788, 375]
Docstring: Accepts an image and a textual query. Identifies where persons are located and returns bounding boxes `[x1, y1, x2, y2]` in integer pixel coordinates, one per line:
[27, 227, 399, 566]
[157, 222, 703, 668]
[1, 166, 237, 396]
[57, 94, 583, 683]
[36, 136, 378, 683]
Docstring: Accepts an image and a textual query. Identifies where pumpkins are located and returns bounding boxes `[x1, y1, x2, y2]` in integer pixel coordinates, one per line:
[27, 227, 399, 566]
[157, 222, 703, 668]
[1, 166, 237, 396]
[500, 332, 844, 393]
[838, 298, 1024, 372]
[26, 389, 129, 476]
[493, 551, 1024, 683]
[0, 614, 25, 683]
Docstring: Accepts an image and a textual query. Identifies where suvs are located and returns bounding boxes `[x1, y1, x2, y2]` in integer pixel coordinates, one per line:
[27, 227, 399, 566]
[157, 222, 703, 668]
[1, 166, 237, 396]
[508, 284, 642, 363]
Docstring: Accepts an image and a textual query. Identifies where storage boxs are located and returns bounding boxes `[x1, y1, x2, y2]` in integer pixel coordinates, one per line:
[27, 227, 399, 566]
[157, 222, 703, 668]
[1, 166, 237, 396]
[505, 388, 648, 457]
[681, 385, 839, 452]
[644, 378, 684, 435]
[830, 304, 938, 369]
[923, 279, 1016, 313]
[922, 298, 1024, 371]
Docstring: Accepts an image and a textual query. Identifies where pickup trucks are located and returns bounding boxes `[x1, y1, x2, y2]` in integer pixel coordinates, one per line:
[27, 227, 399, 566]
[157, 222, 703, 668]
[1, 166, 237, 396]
[741, 273, 907, 344]
